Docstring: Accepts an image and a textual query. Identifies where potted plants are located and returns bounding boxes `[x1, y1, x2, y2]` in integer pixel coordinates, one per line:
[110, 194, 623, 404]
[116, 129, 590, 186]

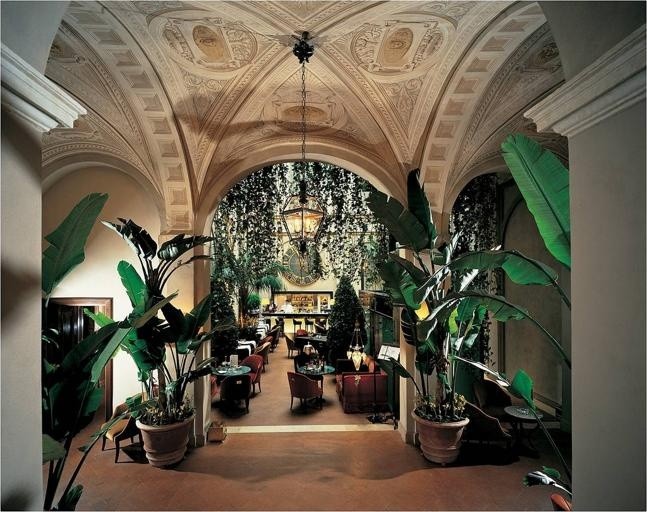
[82, 216, 237, 468]
[351, 165, 491, 465]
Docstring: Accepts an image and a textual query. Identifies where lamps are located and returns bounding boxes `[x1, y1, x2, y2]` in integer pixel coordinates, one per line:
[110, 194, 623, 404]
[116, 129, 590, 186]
[346, 318, 368, 371]
[279, 29, 328, 259]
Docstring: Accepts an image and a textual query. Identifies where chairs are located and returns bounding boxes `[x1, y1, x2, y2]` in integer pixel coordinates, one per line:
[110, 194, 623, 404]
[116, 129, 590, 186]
[459, 378, 521, 463]
[210, 317, 324, 413]
[100, 384, 161, 464]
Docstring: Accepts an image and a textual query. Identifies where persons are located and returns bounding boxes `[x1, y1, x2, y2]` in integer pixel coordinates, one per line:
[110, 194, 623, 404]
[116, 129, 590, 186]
[271, 315, 285, 330]
[297, 344, 319, 368]
[280, 299, 293, 313]
[264, 298, 277, 313]
[315, 317, 327, 334]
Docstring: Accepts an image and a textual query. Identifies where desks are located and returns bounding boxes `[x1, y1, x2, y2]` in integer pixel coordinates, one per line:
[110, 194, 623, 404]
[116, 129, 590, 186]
[503, 404, 544, 459]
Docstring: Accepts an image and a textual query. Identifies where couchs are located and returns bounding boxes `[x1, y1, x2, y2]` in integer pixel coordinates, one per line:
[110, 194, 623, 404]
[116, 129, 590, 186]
[334, 357, 388, 414]
